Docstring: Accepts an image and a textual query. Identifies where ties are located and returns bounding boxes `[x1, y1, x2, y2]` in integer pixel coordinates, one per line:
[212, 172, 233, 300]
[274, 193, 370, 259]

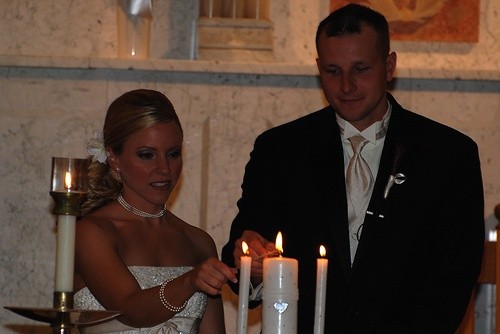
[345, 130, 374, 269]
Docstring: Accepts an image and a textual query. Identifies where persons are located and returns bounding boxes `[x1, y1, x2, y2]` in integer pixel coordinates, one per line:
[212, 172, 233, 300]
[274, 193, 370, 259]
[71, 89, 239, 334]
[219, 2, 485, 334]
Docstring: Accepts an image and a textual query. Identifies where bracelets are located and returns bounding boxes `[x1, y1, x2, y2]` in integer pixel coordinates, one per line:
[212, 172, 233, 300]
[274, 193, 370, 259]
[159, 278, 188, 312]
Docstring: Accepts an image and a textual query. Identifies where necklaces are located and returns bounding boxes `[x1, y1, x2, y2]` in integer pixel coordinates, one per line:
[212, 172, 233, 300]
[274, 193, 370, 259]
[116, 191, 167, 219]
